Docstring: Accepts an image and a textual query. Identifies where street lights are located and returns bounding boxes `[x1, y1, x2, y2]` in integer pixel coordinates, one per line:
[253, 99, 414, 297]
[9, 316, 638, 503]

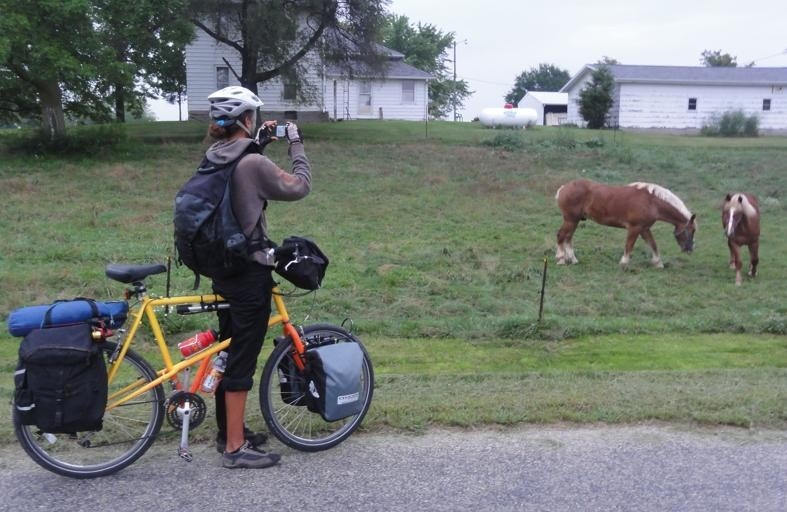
[451, 38, 468, 122]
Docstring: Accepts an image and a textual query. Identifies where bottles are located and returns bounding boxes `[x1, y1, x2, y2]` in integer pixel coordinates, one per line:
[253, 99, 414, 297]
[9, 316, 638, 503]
[177, 330, 228, 355]
[200, 351, 228, 395]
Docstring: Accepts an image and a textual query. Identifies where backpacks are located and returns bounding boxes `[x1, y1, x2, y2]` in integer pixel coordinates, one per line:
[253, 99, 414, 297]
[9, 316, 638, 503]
[272, 333, 311, 407]
[303, 340, 367, 425]
[273, 234, 330, 292]
[172, 144, 277, 282]
[12, 324, 110, 435]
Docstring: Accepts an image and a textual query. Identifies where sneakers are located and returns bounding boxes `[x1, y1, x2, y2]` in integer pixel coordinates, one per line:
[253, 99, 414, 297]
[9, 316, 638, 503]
[221, 439, 284, 470]
[215, 422, 270, 455]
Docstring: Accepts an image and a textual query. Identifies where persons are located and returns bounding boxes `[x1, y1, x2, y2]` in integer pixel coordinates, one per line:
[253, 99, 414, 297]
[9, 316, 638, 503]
[200, 85, 311, 470]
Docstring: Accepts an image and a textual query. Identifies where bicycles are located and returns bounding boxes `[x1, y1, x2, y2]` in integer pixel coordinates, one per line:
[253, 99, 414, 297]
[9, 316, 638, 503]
[11, 237, 376, 481]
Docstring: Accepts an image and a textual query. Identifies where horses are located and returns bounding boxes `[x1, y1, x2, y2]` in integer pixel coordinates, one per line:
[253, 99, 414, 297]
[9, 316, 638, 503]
[721, 191, 761, 286]
[554, 177, 697, 271]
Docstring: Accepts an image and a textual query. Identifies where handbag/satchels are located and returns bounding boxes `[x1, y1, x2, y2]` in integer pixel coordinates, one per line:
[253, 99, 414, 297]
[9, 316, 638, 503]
[7, 297, 130, 337]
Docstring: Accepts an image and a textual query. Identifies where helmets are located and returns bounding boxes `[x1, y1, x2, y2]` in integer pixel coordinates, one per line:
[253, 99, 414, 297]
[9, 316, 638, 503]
[206, 85, 265, 123]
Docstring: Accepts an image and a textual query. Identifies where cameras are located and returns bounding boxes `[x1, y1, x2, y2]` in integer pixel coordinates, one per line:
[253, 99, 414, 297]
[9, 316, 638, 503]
[271, 126, 288, 137]
